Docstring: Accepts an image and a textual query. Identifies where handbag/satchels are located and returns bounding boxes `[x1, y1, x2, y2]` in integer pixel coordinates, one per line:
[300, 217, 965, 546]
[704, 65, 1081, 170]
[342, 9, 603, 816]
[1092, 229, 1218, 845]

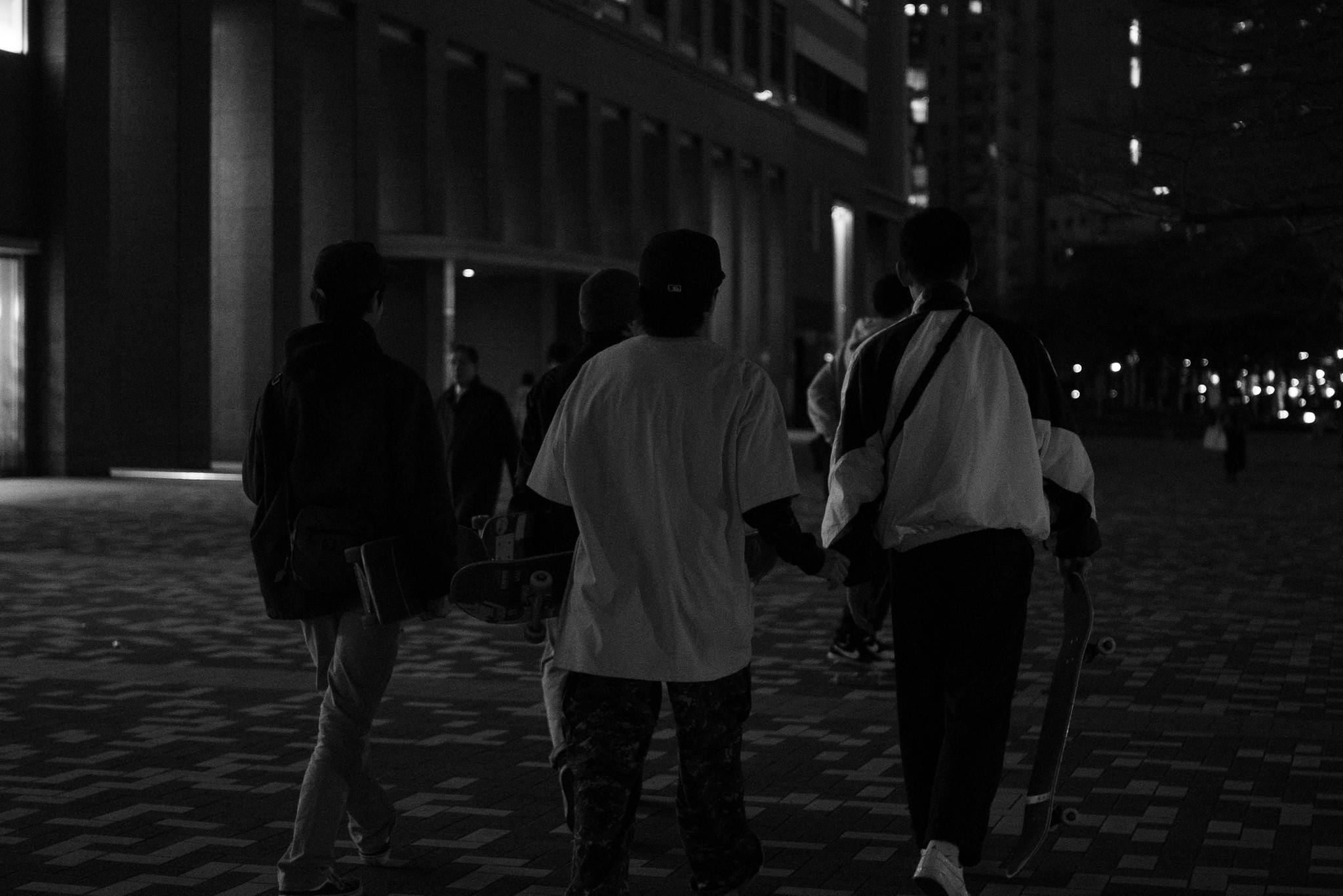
[343, 537, 427, 629]
[1202, 424, 1227, 452]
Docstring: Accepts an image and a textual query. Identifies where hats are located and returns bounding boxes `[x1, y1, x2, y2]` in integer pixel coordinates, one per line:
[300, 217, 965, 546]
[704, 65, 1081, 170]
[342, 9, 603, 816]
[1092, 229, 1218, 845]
[637, 228, 726, 336]
[580, 268, 639, 330]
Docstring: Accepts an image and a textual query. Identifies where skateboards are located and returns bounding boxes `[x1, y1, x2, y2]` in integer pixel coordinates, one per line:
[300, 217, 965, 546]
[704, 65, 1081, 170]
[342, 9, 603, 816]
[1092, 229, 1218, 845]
[344, 514, 489, 631]
[1000, 568, 1098, 880]
[471, 515, 508, 558]
[448, 529, 780, 644]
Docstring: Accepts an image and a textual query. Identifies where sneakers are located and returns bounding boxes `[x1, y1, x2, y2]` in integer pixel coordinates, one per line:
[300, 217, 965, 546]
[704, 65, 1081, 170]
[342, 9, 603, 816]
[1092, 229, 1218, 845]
[911, 849, 967, 896]
[826, 634, 894, 670]
[357, 842, 392, 862]
[278, 869, 362, 896]
[865, 635, 895, 659]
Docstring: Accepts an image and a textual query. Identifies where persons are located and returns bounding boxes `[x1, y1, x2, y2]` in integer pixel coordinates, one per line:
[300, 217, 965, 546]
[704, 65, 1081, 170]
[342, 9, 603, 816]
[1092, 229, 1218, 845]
[524, 228, 847, 896]
[823, 206, 1100, 896]
[806, 276, 899, 671]
[498, 269, 644, 831]
[245, 238, 454, 896]
[435, 341, 514, 619]
[1219, 388, 1247, 478]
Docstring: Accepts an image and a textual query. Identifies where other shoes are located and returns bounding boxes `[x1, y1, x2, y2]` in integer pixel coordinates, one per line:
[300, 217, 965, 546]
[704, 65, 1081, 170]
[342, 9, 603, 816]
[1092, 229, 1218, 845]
[557, 763, 577, 834]
